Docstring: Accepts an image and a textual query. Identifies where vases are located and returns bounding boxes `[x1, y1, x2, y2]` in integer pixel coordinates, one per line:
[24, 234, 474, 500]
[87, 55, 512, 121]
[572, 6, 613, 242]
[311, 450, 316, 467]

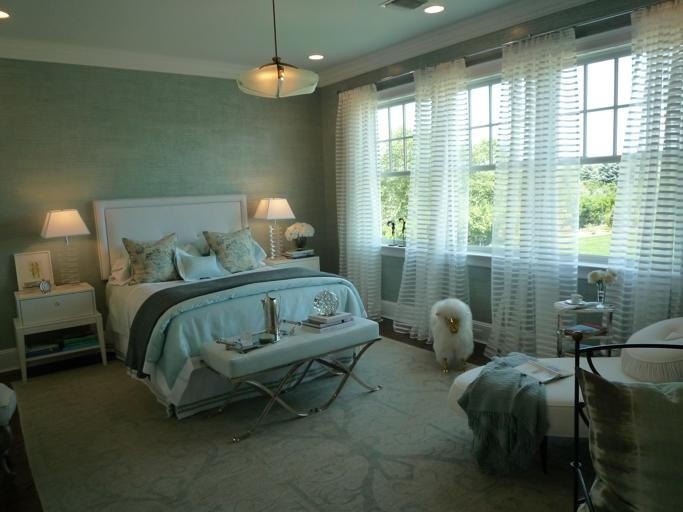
[295, 238, 308, 250]
[595, 285, 607, 312]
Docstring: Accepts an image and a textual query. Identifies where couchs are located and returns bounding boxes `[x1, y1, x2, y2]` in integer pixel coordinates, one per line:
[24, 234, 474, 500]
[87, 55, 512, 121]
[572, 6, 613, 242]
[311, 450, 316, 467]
[451, 319, 682, 481]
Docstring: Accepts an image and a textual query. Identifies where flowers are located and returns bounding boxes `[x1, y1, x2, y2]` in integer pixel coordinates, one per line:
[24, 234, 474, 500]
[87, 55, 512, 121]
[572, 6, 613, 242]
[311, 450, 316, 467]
[286, 222, 315, 239]
[585, 269, 617, 286]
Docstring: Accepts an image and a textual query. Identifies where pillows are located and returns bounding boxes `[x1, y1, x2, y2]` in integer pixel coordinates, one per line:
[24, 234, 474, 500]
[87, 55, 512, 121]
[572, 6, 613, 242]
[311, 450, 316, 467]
[121, 233, 179, 286]
[176, 247, 230, 283]
[623, 319, 683, 382]
[203, 227, 258, 273]
[573, 364, 683, 511]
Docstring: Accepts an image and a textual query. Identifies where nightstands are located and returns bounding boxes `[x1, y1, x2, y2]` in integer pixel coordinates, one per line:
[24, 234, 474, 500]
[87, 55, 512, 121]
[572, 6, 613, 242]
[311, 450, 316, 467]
[264, 255, 320, 272]
[14, 282, 109, 383]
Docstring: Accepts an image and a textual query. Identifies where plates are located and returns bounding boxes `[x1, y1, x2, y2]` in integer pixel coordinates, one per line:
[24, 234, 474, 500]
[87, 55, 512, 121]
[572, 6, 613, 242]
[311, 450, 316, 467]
[564, 299, 586, 305]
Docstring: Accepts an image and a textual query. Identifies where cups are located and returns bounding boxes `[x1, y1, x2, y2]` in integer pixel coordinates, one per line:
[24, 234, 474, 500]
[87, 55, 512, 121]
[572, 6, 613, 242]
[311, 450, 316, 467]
[571, 293, 583, 304]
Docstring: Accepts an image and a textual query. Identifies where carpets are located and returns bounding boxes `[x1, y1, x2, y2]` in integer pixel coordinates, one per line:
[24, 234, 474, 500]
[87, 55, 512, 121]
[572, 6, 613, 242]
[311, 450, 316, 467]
[13, 336, 519, 512]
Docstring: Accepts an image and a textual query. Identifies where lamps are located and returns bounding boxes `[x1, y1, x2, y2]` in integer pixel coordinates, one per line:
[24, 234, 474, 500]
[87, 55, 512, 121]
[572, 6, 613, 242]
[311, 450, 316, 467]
[238, 0, 318, 98]
[254, 198, 295, 260]
[40, 209, 90, 285]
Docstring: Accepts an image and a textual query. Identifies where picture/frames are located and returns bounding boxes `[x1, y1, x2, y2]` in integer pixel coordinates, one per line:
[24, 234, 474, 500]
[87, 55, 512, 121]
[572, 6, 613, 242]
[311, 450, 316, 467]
[14, 250, 52, 290]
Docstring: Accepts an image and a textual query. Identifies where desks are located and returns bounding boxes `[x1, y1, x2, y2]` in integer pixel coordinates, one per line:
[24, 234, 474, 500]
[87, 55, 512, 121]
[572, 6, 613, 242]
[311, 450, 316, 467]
[204, 316, 382, 442]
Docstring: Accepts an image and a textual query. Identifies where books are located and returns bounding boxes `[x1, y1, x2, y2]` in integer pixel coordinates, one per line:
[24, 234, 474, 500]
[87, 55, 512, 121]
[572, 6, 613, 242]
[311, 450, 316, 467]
[563, 322, 608, 340]
[299, 311, 354, 334]
[516, 360, 573, 383]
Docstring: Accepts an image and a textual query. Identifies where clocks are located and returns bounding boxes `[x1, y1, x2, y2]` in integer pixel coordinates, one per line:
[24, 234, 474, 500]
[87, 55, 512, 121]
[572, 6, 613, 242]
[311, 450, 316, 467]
[39, 280, 51, 293]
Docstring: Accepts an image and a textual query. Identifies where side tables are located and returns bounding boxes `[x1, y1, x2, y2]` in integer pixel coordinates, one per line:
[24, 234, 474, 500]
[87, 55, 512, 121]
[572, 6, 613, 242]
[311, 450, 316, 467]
[555, 300, 613, 360]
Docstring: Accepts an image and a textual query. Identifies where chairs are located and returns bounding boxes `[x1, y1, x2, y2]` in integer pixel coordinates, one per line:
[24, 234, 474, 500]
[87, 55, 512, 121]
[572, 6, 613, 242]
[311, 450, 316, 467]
[570, 329, 683, 510]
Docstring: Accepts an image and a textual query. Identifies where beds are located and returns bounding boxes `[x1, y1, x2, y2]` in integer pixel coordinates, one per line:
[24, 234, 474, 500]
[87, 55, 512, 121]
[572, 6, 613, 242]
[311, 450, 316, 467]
[92, 194, 366, 418]
